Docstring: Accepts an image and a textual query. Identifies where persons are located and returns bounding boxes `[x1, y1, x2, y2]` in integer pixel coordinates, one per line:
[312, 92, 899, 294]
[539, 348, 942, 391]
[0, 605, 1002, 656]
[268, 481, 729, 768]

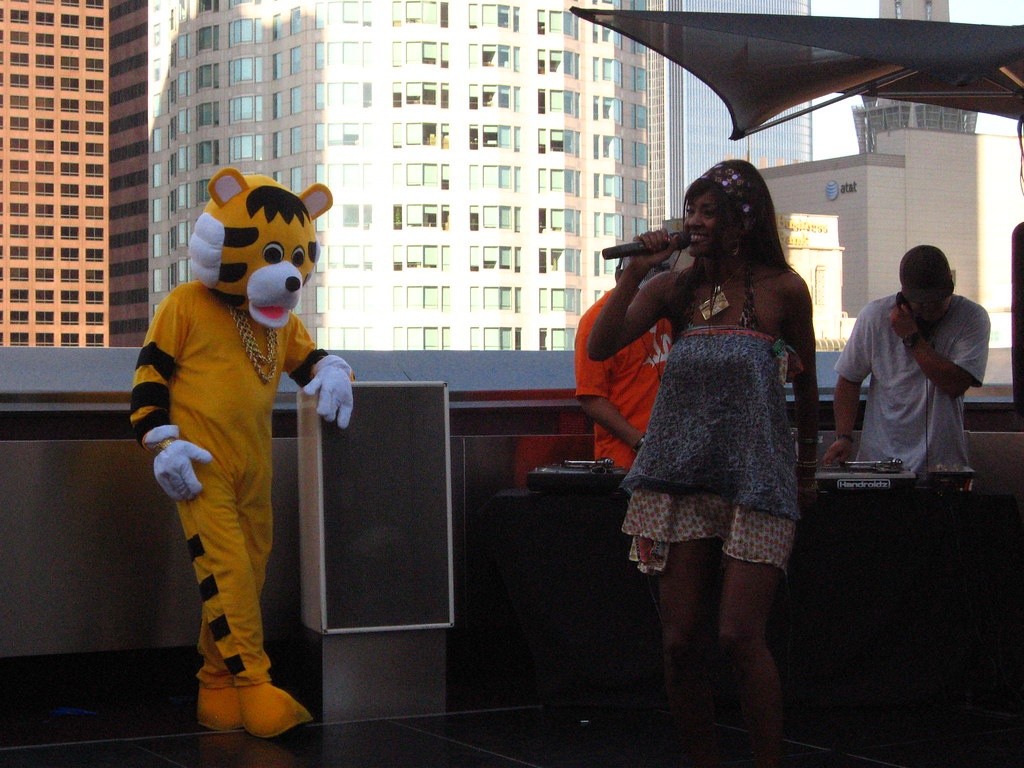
[574, 258, 673, 473]
[822, 244, 991, 480]
[585, 160, 819, 768]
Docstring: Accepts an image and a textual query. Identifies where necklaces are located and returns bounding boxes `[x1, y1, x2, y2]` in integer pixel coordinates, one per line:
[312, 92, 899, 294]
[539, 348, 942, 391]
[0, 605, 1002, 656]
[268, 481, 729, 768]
[226, 304, 278, 383]
[717, 261, 745, 294]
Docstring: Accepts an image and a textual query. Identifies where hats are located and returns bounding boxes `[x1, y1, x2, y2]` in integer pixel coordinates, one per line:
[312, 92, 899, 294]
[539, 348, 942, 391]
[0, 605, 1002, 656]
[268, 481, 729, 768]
[619, 251, 675, 273]
[899, 245, 954, 311]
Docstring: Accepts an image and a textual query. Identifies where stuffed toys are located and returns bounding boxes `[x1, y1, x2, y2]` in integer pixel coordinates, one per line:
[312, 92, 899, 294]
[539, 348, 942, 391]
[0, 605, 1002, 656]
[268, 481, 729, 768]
[131, 167, 356, 738]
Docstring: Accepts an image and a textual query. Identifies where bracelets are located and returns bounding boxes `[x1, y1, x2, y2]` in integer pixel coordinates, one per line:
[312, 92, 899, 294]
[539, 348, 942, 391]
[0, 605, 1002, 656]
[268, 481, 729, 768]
[835, 434, 853, 443]
[803, 477, 816, 480]
[632, 435, 645, 452]
[796, 459, 818, 468]
[796, 436, 818, 444]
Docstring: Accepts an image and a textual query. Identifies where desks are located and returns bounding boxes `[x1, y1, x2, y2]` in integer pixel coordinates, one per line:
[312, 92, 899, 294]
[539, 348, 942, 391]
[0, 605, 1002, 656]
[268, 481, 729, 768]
[473, 490, 1024, 711]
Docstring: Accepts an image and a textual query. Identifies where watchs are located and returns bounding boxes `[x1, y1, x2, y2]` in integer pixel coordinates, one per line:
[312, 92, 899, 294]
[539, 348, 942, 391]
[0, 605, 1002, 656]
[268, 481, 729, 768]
[903, 332, 919, 347]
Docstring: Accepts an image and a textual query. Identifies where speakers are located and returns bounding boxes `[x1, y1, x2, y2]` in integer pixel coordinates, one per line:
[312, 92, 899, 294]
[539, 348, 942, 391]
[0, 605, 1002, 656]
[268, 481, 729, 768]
[297, 381, 455, 635]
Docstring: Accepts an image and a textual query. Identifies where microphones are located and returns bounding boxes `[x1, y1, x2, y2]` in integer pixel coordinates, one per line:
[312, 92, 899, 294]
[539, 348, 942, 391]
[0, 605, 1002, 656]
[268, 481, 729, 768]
[602, 230, 691, 259]
